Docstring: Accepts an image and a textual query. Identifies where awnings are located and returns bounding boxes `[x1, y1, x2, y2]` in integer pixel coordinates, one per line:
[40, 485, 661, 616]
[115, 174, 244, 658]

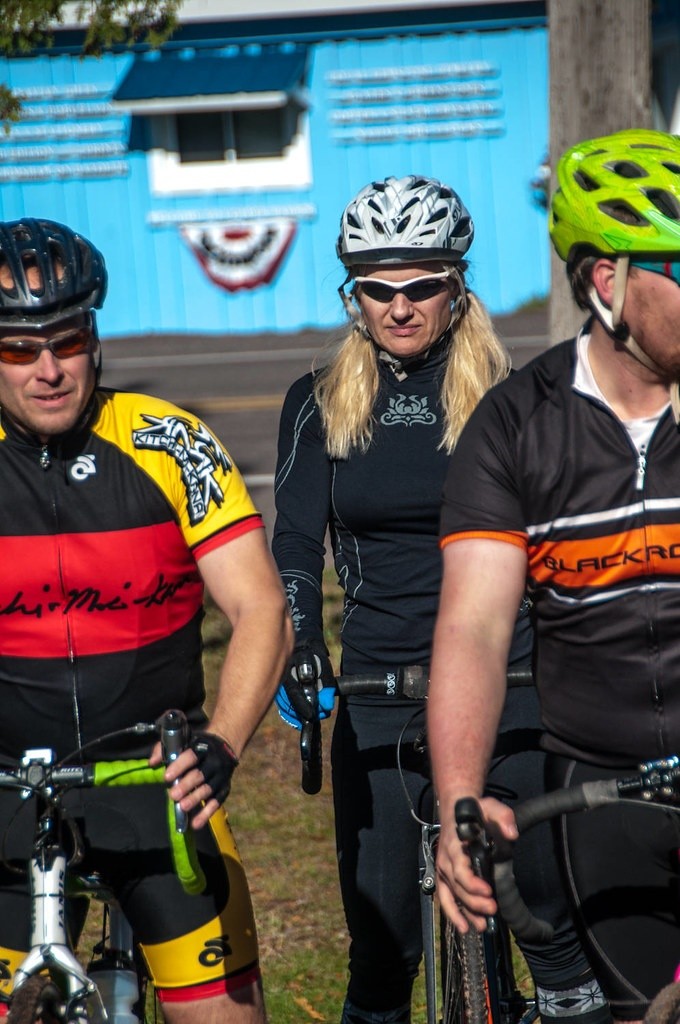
[109, 44, 307, 114]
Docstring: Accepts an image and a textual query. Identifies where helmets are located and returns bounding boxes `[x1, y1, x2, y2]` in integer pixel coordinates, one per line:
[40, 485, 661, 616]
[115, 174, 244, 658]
[336, 176, 474, 268]
[0, 218, 108, 328]
[549, 128, 680, 262]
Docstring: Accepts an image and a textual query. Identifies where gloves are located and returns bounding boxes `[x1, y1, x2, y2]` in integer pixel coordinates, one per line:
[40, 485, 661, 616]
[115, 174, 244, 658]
[275, 647, 337, 732]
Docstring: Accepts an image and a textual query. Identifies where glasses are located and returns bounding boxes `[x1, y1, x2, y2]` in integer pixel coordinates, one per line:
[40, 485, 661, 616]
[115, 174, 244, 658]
[0, 325, 98, 365]
[354, 267, 455, 302]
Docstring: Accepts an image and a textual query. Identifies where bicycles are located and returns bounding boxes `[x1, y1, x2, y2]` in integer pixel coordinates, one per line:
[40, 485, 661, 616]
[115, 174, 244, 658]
[291, 643, 680, 1024]
[1, 706, 210, 1024]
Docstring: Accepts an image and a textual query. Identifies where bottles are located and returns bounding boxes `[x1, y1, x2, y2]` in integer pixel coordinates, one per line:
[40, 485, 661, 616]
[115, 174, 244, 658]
[86, 942, 140, 1024]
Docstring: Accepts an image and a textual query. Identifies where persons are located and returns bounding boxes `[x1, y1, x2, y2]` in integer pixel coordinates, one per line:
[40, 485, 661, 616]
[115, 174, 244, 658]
[427, 129, 680, 1024]
[271, 173, 518, 1024]
[0, 218, 294, 1024]
